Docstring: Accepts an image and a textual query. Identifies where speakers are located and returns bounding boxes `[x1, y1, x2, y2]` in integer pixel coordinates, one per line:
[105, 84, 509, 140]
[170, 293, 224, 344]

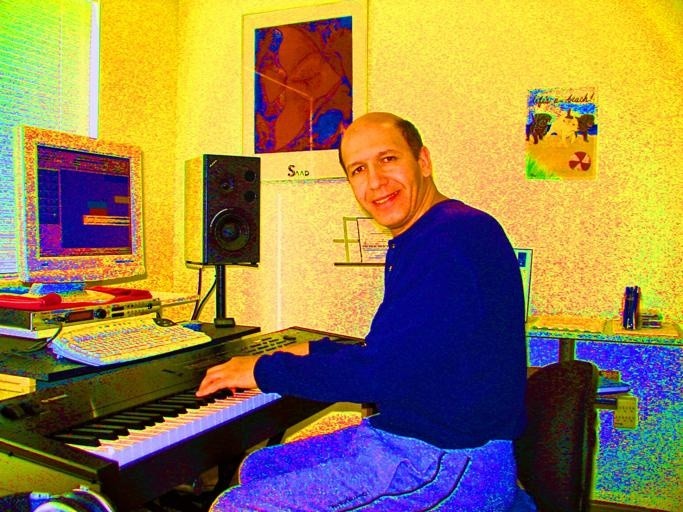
[184, 154, 261, 267]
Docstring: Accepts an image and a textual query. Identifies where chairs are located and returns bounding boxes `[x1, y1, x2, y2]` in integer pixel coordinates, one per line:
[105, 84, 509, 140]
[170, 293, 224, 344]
[514, 360, 599, 512]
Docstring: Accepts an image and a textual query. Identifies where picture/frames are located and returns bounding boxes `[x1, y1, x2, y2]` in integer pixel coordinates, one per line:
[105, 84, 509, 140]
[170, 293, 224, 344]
[514, 246, 533, 322]
[240, 0, 368, 181]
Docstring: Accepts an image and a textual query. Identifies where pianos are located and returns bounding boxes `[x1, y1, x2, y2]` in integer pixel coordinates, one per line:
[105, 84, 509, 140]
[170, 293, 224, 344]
[1, 326, 364, 511]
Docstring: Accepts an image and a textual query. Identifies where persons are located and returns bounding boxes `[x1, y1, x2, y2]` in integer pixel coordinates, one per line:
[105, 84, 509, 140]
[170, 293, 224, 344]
[195, 110, 528, 512]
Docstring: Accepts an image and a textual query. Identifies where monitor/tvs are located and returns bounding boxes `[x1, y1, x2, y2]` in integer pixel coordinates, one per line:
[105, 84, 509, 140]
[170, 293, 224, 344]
[14, 124, 145, 304]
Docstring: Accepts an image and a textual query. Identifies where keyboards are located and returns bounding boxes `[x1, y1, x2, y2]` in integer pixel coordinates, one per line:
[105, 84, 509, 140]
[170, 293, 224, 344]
[51, 316, 211, 366]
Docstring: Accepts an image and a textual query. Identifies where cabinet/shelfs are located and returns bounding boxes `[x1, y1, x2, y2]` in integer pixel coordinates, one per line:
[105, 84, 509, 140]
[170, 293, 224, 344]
[524, 312, 682, 512]
